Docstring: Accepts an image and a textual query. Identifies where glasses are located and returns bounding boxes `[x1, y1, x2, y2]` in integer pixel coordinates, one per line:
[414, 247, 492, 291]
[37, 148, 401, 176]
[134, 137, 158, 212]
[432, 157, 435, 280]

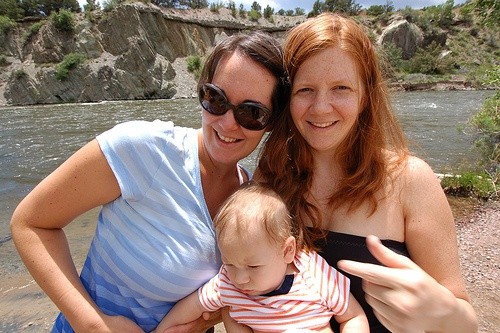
[197, 82, 273, 131]
